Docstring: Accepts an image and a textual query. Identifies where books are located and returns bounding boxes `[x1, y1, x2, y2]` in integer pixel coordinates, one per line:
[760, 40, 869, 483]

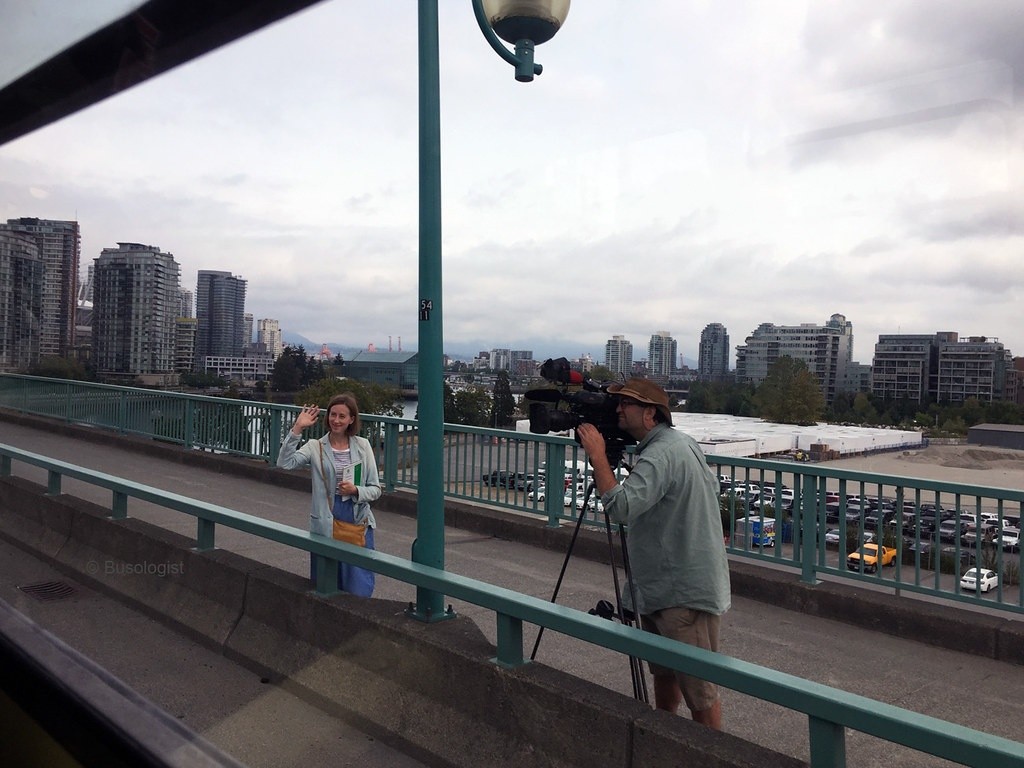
[342, 460, 366, 502]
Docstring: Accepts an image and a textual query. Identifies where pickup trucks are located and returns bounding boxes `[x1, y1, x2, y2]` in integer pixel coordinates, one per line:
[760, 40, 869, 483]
[482, 470, 514, 487]
[845, 543, 896, 573]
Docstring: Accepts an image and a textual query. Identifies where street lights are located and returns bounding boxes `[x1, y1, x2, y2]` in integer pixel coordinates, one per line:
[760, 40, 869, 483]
[411, 0, 573, 621]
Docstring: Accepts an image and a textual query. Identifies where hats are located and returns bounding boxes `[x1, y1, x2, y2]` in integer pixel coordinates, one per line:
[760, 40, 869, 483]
[609, 379, 675, 427]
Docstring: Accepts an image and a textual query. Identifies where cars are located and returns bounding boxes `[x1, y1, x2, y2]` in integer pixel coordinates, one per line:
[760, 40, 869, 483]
[961, 568, 999, 593]
[715, 474, 1021, 553]
[825, 529, 841, 544]
[505, 459, 605, 513]
[864, 532, 876, 543]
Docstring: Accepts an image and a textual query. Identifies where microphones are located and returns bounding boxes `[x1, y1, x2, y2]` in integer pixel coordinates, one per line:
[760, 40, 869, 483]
[524, 388, 562, 402]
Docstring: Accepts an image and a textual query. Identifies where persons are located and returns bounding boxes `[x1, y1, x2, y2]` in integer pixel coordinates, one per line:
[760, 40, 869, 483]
[276, 395, 383, 599]
[576, 377, 731, 734]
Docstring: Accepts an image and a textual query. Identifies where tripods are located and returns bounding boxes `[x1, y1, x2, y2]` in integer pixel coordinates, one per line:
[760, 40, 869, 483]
[529, 440, 649, 706]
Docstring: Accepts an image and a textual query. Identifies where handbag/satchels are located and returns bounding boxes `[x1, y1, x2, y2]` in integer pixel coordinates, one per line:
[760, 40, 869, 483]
[333, 520, 365, 546]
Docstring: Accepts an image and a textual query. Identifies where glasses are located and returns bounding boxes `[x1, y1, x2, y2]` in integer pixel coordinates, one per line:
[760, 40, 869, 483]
[619, 400, 641, 409]
